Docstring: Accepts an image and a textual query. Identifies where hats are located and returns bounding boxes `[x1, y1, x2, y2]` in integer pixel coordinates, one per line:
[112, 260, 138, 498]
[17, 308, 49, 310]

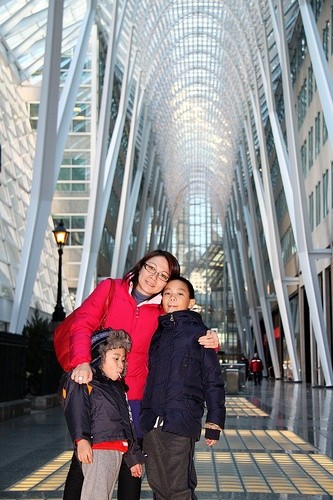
[91, 327, 131, 365]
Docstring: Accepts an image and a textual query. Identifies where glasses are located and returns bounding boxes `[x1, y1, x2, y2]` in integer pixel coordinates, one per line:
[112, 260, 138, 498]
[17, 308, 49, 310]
[143, 263, 170, 282]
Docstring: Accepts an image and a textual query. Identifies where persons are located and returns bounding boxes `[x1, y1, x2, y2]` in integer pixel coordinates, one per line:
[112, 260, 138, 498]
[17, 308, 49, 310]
[239, 356, 250, 381]
[64, 250, 220, 500]
[140, 277, 226, 500]
[64, 327, 146, 500]
[248, 353, 264, 386]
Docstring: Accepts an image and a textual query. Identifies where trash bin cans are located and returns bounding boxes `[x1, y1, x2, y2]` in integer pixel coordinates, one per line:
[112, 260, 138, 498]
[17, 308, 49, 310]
[225, 369, 239, 395]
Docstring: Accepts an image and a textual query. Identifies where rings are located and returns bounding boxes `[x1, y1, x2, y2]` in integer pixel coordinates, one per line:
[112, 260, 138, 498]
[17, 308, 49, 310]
[213, 342, 216, 347]
[78, 376, 83, 380]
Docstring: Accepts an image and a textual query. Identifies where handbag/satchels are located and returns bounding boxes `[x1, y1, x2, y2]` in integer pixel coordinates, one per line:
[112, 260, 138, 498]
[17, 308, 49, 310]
[53, 279, 115, 373]
